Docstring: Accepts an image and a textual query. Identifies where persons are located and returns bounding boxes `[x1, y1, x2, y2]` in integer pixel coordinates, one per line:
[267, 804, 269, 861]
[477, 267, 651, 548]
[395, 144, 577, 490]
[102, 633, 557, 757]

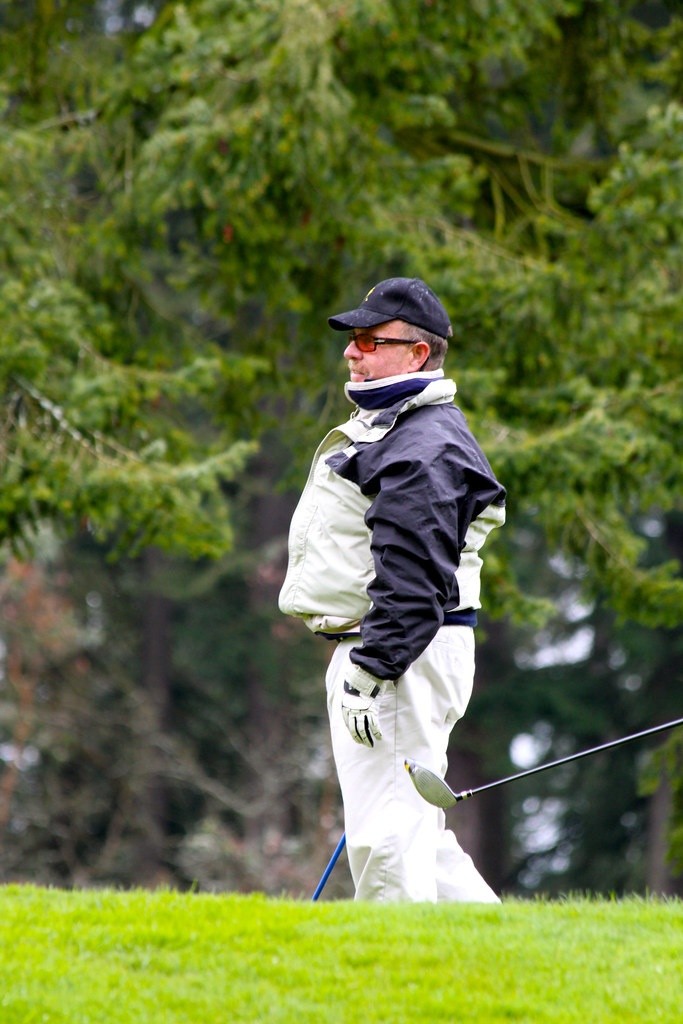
[276, 277, 509, 904]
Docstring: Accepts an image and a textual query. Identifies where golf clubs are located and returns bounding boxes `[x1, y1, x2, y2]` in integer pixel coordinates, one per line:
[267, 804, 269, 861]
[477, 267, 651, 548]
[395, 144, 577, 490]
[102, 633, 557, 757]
[397, 718, 682, 804]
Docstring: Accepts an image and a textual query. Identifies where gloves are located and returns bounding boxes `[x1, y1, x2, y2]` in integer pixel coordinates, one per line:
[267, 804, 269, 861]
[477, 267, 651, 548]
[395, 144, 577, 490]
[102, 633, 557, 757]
[339, 665, 385, 749]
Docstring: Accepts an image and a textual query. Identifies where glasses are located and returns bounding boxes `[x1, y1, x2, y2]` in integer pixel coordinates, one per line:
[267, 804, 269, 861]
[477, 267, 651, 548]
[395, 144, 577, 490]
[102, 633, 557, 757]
[347, 333, 423, 352]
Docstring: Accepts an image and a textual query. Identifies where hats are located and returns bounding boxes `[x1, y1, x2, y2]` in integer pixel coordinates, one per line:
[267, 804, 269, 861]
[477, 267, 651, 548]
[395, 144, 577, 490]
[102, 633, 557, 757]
[327, 276, 451, 339]
